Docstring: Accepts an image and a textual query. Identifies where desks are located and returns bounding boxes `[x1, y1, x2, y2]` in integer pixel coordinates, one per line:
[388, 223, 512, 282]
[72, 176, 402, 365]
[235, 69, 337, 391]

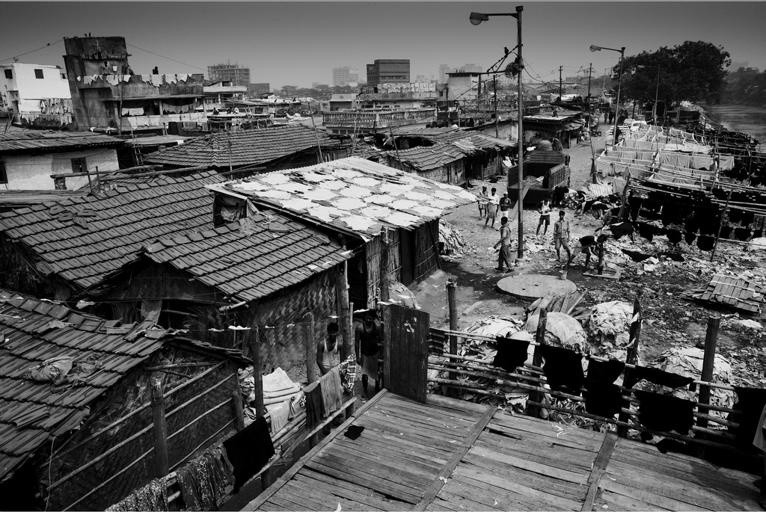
[590, 42, 626, 142]
[469, 5, 525, 268]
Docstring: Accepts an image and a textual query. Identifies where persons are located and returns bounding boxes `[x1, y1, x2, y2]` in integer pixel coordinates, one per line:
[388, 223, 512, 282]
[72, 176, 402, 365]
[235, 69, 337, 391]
[493, 216, 514, 274]
[552, 210, 572, 263]
[604, 108, 629, 124]
[316, 322, 346, 375]
[558, 232, 609, 270]
[484, 187, 501, 229]
[475, 185, 490, 221]
[499, 191, 512, 217]
[535, 196, 553, 235]
